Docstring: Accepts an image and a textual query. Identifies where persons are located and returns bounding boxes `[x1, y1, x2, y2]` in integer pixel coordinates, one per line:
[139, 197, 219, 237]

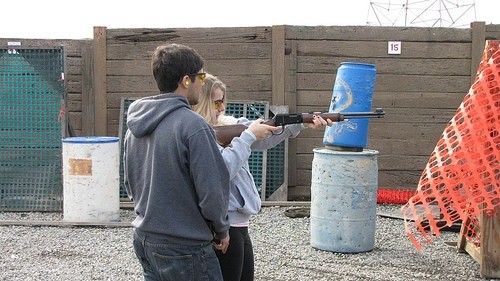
[122, 43, 230, 281]
[188, 73, 333, 281]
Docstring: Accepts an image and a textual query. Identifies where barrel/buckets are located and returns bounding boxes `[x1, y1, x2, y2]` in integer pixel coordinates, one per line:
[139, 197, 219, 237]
[310, 147, 379, 253]
[323, 62, 377, 149]
[62, 135, 121, 222]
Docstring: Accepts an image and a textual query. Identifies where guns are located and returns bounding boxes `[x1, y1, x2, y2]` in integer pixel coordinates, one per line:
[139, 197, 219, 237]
[212, 106, 386, 146]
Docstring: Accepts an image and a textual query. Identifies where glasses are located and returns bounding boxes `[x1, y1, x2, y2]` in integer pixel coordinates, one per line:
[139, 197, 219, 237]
[179, 72, 206, 84]
[211, 95, 224, 109]
